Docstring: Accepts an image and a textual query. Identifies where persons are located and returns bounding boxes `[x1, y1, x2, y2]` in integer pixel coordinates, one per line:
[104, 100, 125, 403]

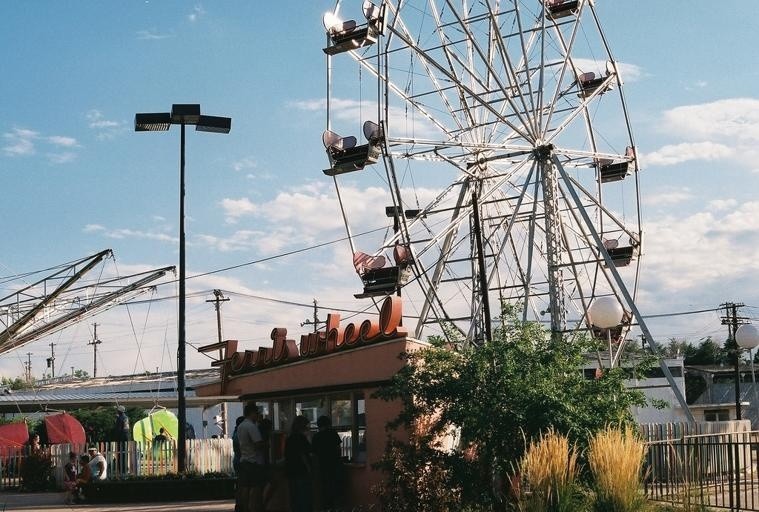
[63, 452, 78, 506]
[284, 414, 315, 511]
[114, 405, 130, 478]
[73, 455, 94, 502]
[232, 415, 245, 511]
[86, 444, 107, 480]
[154, 426, 167, 442]
[257, 418, 273, 508]
[29, 433, 41, 454]
[236, 403, 270, 512]
[312, 415, 345, 511]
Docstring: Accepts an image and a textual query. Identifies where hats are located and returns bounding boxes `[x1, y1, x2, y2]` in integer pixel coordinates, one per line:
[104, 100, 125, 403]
[88, 447, 97, 451]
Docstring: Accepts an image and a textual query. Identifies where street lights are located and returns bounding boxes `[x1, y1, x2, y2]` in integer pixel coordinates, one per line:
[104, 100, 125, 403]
[734, 320, 759, 432]
[130, 98, 236, 478]
[587, 296, 626, 429]
